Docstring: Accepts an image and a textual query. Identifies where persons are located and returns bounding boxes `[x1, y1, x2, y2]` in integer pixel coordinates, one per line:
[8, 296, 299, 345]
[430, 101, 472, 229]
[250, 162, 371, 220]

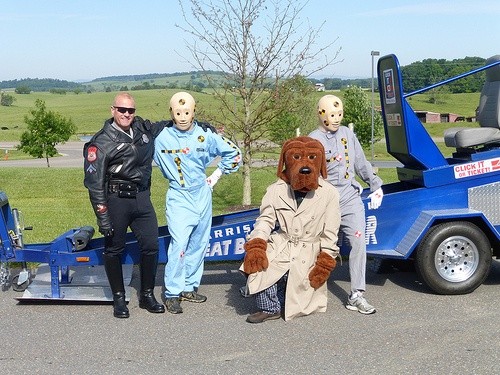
[153, 92, 242, 314]
[307, 94, 383, 315]
[83, 92, 218, 317]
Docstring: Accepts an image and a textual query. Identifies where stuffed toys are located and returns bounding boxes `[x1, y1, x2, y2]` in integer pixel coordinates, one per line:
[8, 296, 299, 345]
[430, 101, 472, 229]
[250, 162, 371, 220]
[239, 136, 341, 324]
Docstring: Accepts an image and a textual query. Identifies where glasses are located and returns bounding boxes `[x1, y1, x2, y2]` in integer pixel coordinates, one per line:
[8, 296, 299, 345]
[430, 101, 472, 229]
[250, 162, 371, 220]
[113, 106, 136, 114]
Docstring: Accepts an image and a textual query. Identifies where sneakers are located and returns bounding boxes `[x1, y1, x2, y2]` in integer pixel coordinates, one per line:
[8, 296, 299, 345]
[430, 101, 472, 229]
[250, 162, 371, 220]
[180, 290, 208, 303]
[166, 298, 183, 314]
[345, 295, 376, 315]
[239, 286, 251, 298]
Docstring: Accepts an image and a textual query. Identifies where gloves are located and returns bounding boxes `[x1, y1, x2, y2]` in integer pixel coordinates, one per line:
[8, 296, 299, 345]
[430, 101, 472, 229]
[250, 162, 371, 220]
[309, 251, 336, 291]
[99, 223, 119, 237]
[205, 168, 222, 193]
[366, 188, 383, 210]
[196, 120, 217, 133]
[244, 238, 269, 274]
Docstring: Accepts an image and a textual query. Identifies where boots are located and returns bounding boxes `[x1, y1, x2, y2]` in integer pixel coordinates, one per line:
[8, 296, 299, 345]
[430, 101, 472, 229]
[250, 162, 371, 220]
[139, 254, 165, 312]
[102, 252, 129, 318]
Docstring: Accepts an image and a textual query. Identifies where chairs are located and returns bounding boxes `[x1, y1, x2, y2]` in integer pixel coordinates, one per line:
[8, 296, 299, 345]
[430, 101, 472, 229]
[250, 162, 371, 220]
[443, 54, 500, 149]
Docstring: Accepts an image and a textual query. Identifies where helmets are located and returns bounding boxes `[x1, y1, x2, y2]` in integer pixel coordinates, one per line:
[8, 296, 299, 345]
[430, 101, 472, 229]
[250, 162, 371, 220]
[316, 94, 343, 133]
[169, 92, 196, 130]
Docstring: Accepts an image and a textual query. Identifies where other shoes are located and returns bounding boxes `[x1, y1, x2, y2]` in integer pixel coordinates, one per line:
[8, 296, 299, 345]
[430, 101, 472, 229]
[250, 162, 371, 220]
[246, 311, 281, 323]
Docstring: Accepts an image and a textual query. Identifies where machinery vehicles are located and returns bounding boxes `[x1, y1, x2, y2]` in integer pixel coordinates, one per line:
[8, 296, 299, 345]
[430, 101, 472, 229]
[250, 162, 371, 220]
[0, 52, 500, 304]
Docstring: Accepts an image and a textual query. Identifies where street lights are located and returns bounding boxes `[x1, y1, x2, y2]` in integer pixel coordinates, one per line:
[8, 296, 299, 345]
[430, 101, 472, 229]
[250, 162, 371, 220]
[370, 50, 381, 167]
